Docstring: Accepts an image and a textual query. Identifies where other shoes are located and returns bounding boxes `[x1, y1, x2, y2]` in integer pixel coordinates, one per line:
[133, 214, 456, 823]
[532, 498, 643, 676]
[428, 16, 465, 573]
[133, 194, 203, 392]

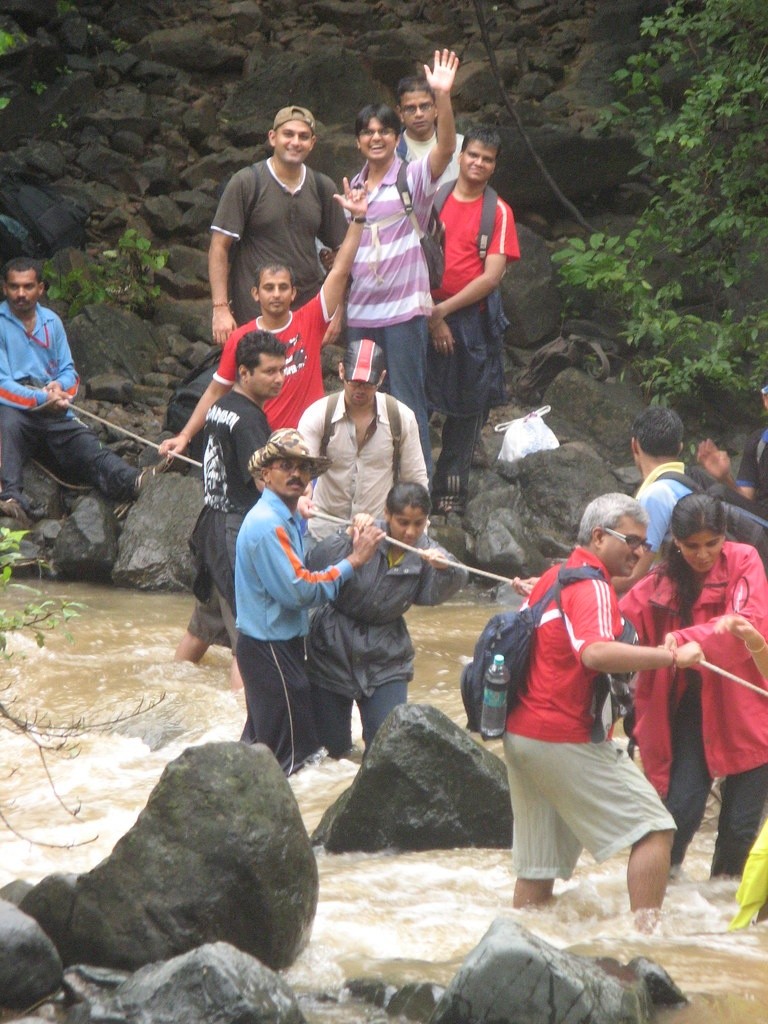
[434, 505, 465, 515]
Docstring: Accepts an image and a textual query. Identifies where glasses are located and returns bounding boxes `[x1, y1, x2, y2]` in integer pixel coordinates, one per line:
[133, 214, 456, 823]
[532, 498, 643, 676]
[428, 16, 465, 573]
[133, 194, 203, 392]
[603, 527, 653, 554]
[401, 103, 434, 115]
[346, 381, 378, 389]
[269, 461, 313, 472]
[359, 127, 396, 137]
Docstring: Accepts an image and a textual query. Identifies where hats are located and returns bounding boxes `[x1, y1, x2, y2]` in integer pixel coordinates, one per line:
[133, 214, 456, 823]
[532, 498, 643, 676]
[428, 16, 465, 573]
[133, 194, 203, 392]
[343, 339, 384, 384]
[248, 427, 333, 481]
[273, 105, 315, 134]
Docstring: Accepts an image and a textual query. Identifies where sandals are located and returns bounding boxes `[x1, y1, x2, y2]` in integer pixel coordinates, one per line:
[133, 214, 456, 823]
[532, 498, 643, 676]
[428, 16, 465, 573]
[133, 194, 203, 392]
[131, 457, 174, 502]
[0, 501, 28, 523]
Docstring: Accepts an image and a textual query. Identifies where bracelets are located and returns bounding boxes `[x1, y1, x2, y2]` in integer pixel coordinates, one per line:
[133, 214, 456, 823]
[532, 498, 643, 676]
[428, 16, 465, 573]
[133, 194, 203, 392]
[745, 640, 766, 653]
[670, 648, 677, 671]
[349, 216, 366, 223]
[212, 303, 228, 307]
[180, 432, 191, 443]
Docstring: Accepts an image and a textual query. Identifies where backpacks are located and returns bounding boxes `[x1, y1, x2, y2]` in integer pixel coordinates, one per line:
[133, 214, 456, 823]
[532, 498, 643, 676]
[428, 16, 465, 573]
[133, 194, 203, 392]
[396, 161, 444, 289]
[460, 566, 607, 740]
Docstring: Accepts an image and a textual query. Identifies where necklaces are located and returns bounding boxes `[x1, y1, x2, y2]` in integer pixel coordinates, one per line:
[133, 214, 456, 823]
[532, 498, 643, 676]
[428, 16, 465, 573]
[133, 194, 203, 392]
[273, 160, 301, 187]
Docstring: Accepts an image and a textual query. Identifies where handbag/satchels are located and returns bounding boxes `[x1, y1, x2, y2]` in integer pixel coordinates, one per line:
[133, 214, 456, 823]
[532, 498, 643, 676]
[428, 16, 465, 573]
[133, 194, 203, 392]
[167, 347, 223, 434]
[494, 404, 560, 462]
[509, 336, 579, 402]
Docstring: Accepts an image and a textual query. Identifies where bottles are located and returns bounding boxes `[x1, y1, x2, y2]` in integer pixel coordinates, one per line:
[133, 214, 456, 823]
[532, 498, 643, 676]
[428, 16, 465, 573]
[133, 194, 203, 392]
[481, 655, 510, 736]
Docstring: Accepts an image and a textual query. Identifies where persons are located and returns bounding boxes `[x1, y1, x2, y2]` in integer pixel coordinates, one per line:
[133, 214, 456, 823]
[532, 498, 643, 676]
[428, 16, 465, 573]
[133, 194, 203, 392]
[304, 480, 469, 762]
[173, 330, 287, 685]
[712, 613, 768, 928]
[319, 48, 460, 463]
[234, 427, 387, 777]
[207, 106, 348, 346]
[512, 408, 697, 597]
[298, 339, 431, 548]
[392, 76, 464, 187]
[502, 490, 705, 931]
[1, 259, 193, 514]
[379, 124, 522, 516]
[616, 491, 768, 879]
[698, 375, 768, 507]
[159, 177, 369, 459]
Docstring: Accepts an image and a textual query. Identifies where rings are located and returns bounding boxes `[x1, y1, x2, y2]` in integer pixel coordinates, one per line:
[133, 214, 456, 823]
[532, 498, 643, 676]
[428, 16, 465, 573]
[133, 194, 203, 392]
[442, 341, 447, 344]
